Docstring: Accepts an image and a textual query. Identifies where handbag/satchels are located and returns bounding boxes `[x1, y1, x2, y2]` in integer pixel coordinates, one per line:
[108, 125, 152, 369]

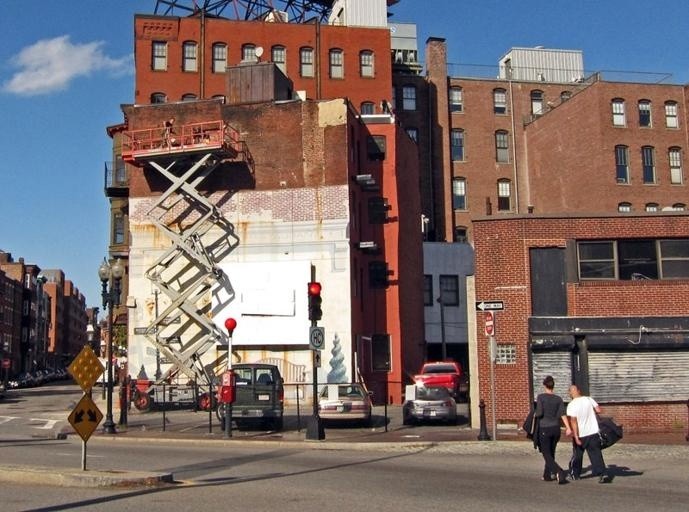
[595, 412, 623, 450]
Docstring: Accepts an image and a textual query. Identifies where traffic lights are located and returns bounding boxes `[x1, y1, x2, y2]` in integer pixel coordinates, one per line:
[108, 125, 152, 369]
[307, 282, 324, 321]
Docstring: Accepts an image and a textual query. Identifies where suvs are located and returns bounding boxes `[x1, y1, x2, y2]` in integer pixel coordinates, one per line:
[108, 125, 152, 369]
[216, 364, 285, 431]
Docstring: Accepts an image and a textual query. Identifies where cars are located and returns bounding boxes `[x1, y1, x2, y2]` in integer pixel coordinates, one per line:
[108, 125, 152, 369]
[318, 384, 373, 427]
[5, 367, 76, 390]
[402, 386, 457, 426]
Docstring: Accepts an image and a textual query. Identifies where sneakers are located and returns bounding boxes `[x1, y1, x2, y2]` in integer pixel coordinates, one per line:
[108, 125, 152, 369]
[598, 475, 610, 484]
[555, 470, 568, 484]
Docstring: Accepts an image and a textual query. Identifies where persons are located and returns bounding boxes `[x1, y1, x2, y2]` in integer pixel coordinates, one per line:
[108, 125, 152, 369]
[566, 384, 612, 484]
[156, 117, 178, 150]
[533, 374, 572, 485]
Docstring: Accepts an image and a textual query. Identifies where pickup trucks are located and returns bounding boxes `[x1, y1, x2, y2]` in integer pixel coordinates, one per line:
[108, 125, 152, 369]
[413, 360, 468, 402]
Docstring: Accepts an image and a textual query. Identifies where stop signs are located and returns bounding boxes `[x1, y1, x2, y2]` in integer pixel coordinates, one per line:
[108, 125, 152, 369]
[2, 359, 10, 369]
[485, 312, 495, 336]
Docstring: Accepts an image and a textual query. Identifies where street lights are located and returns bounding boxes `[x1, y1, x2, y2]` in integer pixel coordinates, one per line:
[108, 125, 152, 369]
[98, 256, 124, 434]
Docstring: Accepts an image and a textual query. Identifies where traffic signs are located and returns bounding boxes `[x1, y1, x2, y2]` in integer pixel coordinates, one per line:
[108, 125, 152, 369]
[475, 301, 504, 312]
